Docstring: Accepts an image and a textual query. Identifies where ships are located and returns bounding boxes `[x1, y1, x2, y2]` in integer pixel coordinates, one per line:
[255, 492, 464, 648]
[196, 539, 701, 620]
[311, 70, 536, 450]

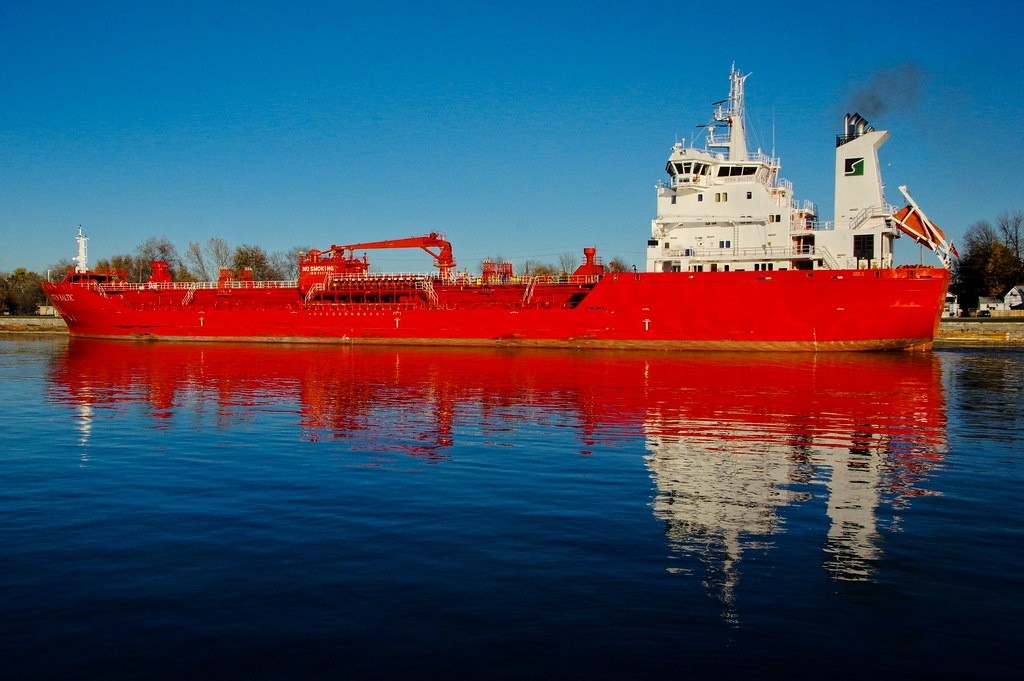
[39, 59, 960, 353]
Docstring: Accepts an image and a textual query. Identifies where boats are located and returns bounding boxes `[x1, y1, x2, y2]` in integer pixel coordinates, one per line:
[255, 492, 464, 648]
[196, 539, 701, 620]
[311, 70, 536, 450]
[891, 205, 945, 251]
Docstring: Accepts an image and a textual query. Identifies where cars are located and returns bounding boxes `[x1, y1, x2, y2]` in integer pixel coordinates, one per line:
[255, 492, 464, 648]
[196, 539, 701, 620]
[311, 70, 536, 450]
[977, 309, 992, 317]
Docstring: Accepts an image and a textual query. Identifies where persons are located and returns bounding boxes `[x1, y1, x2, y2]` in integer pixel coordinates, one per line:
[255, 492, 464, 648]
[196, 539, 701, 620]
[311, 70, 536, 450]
[491, 273, 509, 285]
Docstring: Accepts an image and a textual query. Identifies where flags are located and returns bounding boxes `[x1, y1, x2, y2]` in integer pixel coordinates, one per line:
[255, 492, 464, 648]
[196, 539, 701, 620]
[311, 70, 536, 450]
[949, 242, 960, 258]
